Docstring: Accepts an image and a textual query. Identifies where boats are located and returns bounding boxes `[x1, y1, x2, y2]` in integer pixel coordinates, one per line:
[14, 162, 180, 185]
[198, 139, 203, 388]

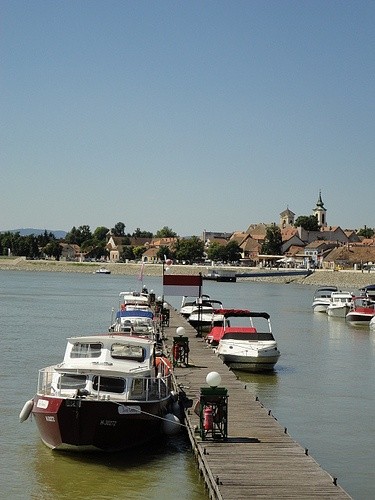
[19, 333, 191, 451]
[216, 312, 280, 374]
[108, 291, 163, 341]
[180, 294, 257, 347]
[311, 284, 375, 325]
[96, 266, 111, 274]
